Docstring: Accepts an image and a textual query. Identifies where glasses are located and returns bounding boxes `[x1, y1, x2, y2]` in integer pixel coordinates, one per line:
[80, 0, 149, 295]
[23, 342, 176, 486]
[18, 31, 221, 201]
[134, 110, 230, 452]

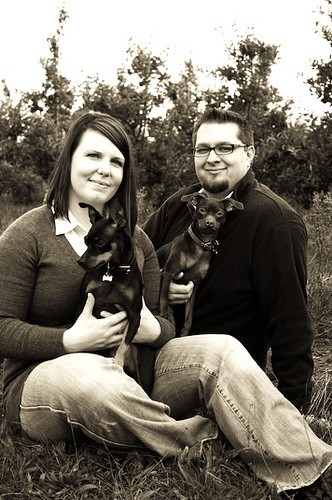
[190, 144, 246, 156]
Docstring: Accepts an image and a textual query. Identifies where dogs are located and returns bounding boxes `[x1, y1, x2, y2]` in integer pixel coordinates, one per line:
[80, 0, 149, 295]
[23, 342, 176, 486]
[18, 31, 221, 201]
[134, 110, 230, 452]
[155, 193, 244, 339]
[77, 202, 156, 400]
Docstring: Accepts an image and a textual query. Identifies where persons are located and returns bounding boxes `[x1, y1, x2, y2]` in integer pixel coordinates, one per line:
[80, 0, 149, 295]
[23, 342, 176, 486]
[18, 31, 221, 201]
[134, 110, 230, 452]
[136, 109, 314, 414]
[0, 113, 330, 500]
